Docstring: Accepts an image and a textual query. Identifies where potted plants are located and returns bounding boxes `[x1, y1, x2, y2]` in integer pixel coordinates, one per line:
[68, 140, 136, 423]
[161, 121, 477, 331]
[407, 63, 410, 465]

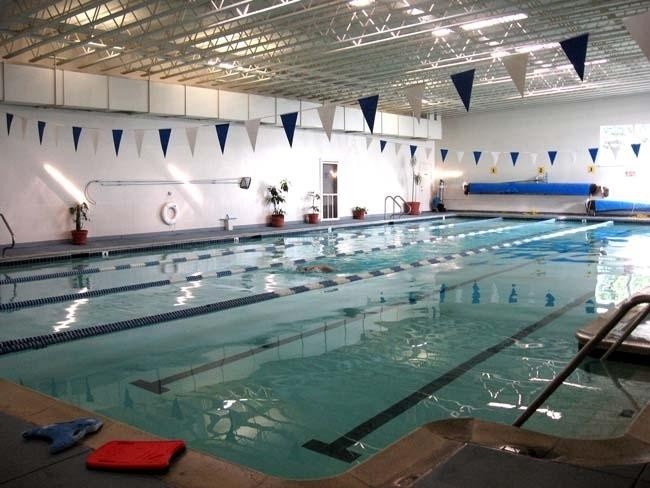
[266, 180, 289, 227]
[351, 206, 368, 219]
[69, 202, 92, 244]
[403, 155, 423, 215]
[304, 192, 322, 224]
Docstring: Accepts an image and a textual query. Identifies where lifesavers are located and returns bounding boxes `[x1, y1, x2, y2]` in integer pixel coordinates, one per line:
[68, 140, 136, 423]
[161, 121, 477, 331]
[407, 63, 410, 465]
[162, 203, 177, 222]
